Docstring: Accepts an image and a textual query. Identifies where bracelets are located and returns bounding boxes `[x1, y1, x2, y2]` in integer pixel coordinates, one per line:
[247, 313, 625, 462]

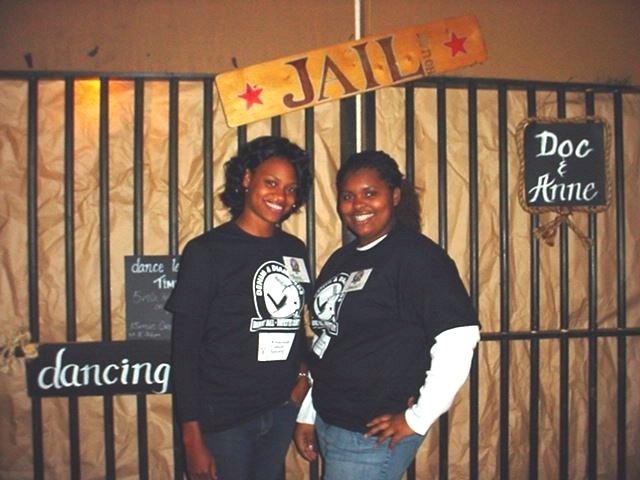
[297, 371, 312, 378]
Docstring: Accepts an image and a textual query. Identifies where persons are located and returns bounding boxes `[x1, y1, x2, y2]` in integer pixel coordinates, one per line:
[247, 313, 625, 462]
[165, 134, 314, 479]
[292, 148, 482, 479]
